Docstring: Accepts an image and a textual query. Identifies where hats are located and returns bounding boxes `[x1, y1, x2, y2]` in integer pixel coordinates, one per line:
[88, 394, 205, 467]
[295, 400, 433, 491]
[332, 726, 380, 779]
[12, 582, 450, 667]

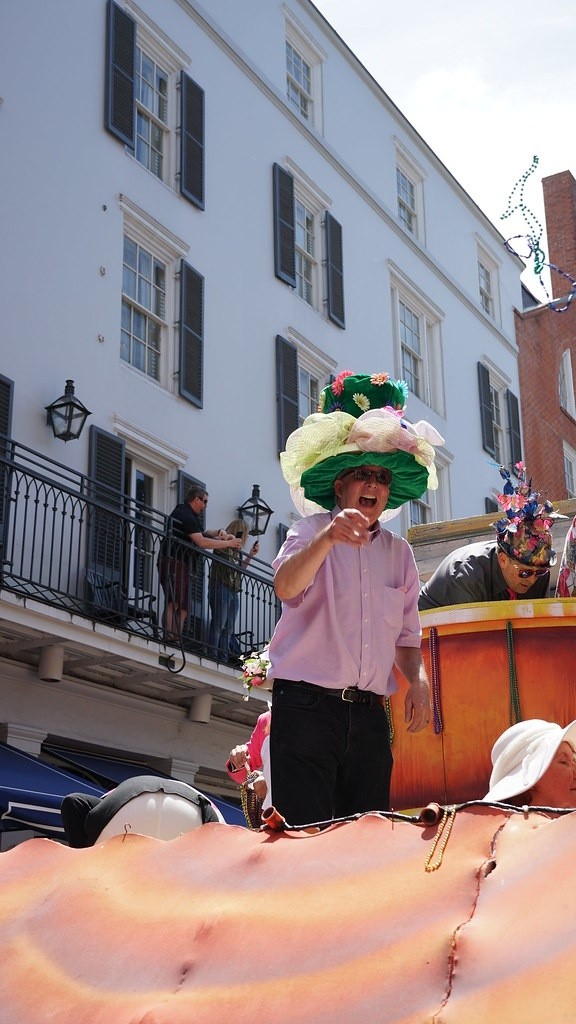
[489, 461, 569, 568]
[480, 718, 575, 803]
[239, 641, 274, 702]
[279, 371, 445, 522]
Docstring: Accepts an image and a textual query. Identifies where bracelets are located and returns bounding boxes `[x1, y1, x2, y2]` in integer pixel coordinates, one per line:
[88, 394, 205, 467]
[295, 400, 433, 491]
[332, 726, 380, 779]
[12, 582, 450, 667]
[218, 528, 224, 535]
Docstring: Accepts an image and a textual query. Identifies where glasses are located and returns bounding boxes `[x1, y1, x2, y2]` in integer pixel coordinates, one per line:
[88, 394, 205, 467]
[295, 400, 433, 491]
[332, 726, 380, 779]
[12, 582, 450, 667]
[340, 468, 391, 485]
[509, 557, 548, 578]
[198, 496, 207, 505]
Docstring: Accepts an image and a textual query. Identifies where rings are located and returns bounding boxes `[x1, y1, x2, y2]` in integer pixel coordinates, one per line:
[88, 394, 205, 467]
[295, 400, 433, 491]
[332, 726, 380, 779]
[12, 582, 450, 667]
[425, 720, 429, 723]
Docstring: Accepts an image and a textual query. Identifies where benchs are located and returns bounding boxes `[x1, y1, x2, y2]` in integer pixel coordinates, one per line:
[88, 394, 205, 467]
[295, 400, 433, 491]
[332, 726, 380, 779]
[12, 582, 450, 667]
[163, 608, 252, 675]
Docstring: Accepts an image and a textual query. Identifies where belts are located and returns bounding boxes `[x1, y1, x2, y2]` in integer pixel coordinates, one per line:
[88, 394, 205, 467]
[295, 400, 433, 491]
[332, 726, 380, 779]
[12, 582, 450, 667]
[287, 680, 384, 706]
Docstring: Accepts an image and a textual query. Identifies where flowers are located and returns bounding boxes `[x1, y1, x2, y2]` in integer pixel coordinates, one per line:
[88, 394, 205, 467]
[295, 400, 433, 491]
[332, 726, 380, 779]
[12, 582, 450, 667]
[315, 369, 410, 421]
[238, 644, 274, 700]
[491, 460, 563, 568]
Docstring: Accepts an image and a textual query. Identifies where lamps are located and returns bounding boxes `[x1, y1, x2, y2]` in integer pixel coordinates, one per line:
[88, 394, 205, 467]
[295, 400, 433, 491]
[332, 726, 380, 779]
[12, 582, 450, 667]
[236, 484, 276, 535]
[44, 378, 94, 443]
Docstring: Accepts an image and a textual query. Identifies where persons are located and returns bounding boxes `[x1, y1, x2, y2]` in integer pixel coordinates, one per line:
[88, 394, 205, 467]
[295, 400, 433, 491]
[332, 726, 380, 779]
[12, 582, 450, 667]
[267, 466, 432, 826]
[156, 485, 241, 648]
[225, 711, 272, 811]
[482, 719, 576, 816]
[209, 519, 259, 662]
[418, 540, 550, 612]
[60, 776, 227, 848]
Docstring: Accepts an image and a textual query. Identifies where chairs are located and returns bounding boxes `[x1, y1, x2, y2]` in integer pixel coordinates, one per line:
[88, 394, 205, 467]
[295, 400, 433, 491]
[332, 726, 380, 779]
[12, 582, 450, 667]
[83, 568, 157, 640]
